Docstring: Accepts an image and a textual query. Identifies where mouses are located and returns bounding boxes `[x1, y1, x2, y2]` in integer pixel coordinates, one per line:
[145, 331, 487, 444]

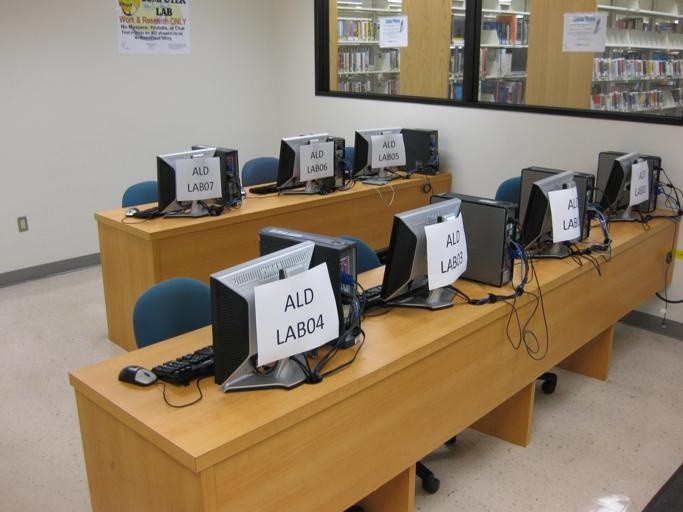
[125, 207, 139, 217]
[118, 365, 159, 387]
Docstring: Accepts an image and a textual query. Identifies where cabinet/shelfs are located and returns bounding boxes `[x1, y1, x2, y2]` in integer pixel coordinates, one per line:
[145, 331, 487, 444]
[329, 1, 399, 94]
[530, 1, 683, 114]
[400, 3, 528, 104]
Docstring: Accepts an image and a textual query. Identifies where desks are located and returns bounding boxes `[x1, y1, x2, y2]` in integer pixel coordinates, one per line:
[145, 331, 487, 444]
[67, 201, 680, 510]
[94, 165, 453, 355]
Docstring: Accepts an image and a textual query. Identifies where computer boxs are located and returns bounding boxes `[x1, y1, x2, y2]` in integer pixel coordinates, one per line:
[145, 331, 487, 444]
[259, 225, 359, 350]
[400, 127, 439, 177]
[191, 144, 240, 207]
[327, 139, 345, 187]
[524, 164, 595, 244]
[429, 192, 518, 288]
[596, 151, 661, 213]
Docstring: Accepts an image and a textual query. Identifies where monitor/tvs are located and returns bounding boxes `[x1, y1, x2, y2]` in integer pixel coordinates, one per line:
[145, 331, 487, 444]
[210, 239, 325, 393]
[519, 172, 580, 262]
[276, 135, 335, 194]
[381, 198, 464, 311]
[595, 150, 651, 223]
[353, 130, 409, 186]
[156, 148, 227, 219]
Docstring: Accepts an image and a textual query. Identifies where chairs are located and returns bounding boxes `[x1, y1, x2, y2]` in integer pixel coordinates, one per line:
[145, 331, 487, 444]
[122, 180, 162, 208]
[536, 373, 559, 394]
[334, 237, 462, 494]
[132, 277, 213, 350]
[343, 147, 356, 173]
[241, 157, 280, 186]
[495, 177, 522, 202]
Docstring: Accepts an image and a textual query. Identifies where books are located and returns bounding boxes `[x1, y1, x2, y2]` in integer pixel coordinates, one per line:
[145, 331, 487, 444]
[336, 20, 380, 43]
[479, 79, 524, 103]
[592, 58, 683, 80]
[591, 92, 683, 112]
[594, 82, 610, 92]
[449, 80, 463, 99]
[338, 46, 398, 73]
[480, 48, 512, 77]
[451, 17, 466, 47]
[483, 13, 529, 46]
[338, 74, 400, 95]
[612, 48, 648, 59]
[653, 49, 683, 59]
[450, 49, 464, 79]
[614, 17, 683, 33]
[612, 81, 683, 92]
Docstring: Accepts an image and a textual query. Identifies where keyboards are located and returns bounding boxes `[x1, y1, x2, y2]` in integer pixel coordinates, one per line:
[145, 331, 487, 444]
[355, 284, 383, 307]
[152, 344, 214, 387]
[134, 206, 162, 222]
[249, 183, 276, 195]
